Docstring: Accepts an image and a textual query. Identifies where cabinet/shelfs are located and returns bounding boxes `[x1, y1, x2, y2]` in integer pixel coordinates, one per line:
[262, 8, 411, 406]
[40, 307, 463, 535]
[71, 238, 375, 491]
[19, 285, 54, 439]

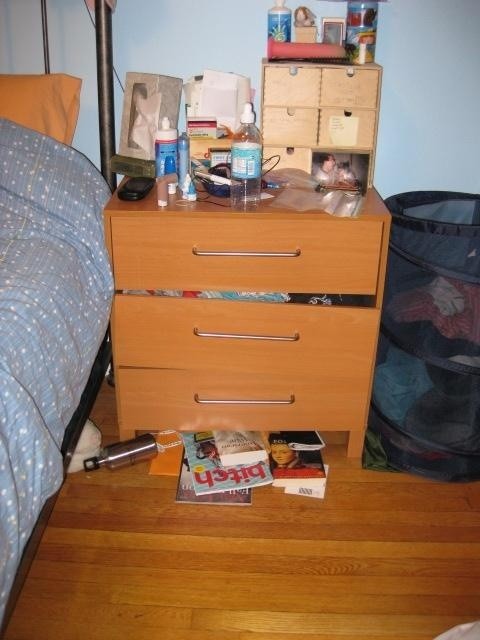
[260, 58, 382, 191]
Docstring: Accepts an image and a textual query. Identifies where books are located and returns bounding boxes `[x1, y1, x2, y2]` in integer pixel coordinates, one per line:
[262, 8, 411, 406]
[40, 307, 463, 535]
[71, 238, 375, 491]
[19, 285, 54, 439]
[148, 429, 330, 506]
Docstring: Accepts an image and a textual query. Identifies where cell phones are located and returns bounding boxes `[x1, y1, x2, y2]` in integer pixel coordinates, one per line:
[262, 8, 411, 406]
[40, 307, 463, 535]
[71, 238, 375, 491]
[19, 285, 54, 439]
[118, 176, 155, 201]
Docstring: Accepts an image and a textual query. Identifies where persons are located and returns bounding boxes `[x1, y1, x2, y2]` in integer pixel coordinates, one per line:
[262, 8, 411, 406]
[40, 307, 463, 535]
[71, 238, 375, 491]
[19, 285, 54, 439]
[313, 153, 337, 186]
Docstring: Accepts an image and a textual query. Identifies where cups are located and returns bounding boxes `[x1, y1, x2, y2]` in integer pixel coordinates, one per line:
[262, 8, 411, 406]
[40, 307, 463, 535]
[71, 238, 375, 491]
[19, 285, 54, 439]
[346, 2, 375, 64]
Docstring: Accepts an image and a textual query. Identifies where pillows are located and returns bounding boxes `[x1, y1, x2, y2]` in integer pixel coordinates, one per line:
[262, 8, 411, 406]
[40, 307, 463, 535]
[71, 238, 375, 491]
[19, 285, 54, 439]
[0, 72, 83, 147]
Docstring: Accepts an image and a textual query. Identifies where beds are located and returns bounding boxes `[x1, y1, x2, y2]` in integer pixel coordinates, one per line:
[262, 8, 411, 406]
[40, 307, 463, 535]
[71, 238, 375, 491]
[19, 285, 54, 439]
[0, 0, 116, 640]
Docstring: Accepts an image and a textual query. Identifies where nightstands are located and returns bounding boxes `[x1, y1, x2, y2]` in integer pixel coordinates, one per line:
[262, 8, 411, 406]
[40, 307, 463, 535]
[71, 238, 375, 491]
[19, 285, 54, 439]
[104, 179, 391, 457]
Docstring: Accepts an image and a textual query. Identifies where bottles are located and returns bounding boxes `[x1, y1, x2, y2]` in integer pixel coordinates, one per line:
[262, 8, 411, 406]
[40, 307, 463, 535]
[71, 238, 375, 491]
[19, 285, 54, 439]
[83, 432, 159, 472]
[267, 0, 290, 42]
[230, 103, 262, 209]
[154, 115, 198, 207]
